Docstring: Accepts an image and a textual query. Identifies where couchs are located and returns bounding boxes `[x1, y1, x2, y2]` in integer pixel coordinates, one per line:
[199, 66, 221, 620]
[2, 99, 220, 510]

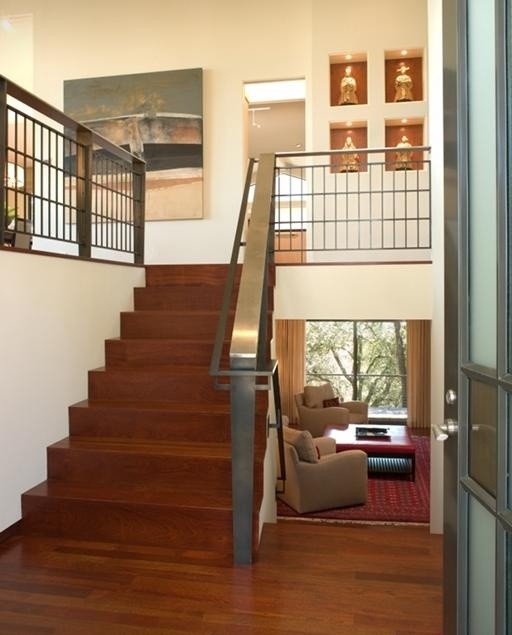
[283, 426, 368, 514]
[293, 382, 369, 437]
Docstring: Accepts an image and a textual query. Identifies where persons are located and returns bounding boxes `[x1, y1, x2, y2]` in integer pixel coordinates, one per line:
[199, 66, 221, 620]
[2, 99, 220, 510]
[392, 64, 415, 101]
[392, 134, 413, 169]
[339, 135, 360, 172]
[337, 63, 359, 105]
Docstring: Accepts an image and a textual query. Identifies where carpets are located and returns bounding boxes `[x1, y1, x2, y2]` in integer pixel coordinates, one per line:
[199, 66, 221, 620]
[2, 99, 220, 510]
[278, 435, 430, 523]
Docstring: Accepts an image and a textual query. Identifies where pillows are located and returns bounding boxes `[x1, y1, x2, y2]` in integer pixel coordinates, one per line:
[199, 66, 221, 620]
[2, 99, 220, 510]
[303, 383, 335, 407]
[282, 425, 319, 462]
[323, 398, 339, 408]
[316, 446, 320, 460]
